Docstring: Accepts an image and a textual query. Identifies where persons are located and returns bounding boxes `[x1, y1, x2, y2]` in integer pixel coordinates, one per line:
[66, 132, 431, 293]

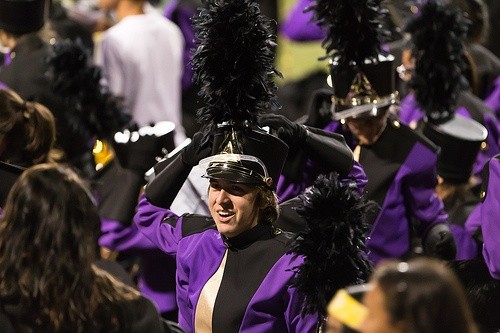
[92, 0, 186, 136]
[481, 76, 500, 153]
[275, 0, 333, 44]
[0, 0, 49, 66]
[391, 69, 482, 131]
[463, 153, 500, 282]
[0, 89, 56, 169]
[236, 112, 356, 206]
[466, 280, 500, 332]
[163, 0, 204, 95]
[325, 284, 378, 333]
[97, 131, 180, 324]
[275, 59, 458, 284]
[133, 120, 327, 333]
[0, 46, 50, 90]
[363, 257, 478, 332]
[0, 162, 186, 333]
[0, 161, 26, 220]
[420, 106, 489, 226]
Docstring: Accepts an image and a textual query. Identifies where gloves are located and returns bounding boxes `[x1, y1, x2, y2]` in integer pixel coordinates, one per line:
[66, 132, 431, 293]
[145, 130, 223, 207]
[302, 88, 334, 128]
[96, 134, 158, 223]
[257, 110, 354, 176]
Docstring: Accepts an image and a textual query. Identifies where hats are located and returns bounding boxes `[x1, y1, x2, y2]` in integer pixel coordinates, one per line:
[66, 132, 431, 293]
[0, 0, 45, 32]
[301, 0, 398, 120]
[397, 0, 486, 186]
[284, 171, 376, 333]
[43, 41, 176, 167]
[190, 0, 290, 191]
